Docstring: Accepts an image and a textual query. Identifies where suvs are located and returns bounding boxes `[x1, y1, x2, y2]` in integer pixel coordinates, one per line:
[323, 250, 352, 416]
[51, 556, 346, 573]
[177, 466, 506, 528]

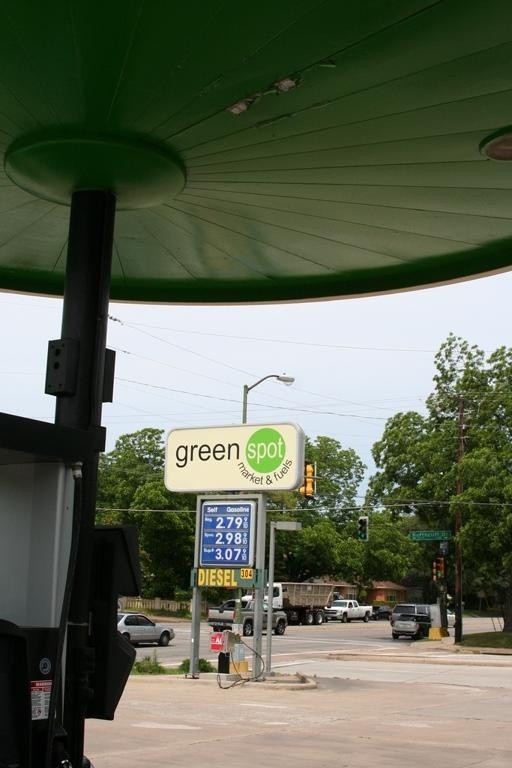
[325, 599, 456, 641]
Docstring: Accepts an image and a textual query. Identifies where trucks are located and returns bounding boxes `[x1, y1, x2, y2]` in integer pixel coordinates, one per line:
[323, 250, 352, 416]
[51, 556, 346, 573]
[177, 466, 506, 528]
[242, 581, 334, 626]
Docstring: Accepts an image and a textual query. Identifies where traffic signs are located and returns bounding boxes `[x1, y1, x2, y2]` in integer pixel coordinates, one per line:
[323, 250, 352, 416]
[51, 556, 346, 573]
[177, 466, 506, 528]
[407, 530, 452, 542]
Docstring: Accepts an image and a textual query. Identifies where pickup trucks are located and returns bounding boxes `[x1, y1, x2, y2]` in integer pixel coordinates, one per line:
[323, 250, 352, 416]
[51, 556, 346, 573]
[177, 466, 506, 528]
[207, 599, 288, 636]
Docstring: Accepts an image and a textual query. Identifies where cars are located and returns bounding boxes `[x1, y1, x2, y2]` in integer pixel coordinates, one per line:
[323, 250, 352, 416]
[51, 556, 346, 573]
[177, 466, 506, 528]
[115, 611, 176, 648]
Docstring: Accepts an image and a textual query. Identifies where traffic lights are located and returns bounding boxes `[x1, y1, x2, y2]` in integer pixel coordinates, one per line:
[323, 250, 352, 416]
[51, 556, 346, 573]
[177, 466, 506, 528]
[436, 558, 444, 579]
[432, 561, 437, 582]
[359, 516, 369, 542]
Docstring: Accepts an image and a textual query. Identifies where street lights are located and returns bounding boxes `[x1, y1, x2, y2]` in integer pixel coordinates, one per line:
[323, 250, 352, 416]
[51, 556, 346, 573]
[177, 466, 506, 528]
[241, 371, 297, 428]
